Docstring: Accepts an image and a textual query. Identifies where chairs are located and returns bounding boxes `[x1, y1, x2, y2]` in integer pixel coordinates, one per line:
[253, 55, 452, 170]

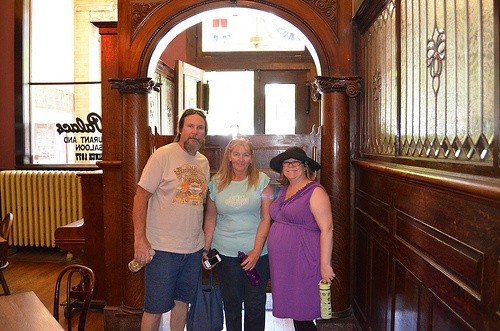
[53, 264, 96, 331]
[0, 212, 14, 295]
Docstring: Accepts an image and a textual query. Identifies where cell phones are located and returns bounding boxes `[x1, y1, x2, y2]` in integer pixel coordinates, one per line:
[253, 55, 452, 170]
[204, 254, 222, 268]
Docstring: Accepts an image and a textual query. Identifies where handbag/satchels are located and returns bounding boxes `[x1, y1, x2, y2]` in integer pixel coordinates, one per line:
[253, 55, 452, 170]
[186, 261, 224, 331]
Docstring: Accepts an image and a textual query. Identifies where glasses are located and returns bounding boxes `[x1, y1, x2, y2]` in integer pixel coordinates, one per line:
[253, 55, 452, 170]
[281, 161, 305, 167]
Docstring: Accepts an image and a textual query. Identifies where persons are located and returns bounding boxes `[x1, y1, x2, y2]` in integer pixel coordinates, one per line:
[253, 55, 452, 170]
[203, 137, 275, 331]
[267, 145, 337, 331]
[132, 108, 210, 331]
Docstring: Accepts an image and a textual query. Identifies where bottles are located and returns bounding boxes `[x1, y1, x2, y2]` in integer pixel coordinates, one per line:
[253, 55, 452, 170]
[319, 280, 332, 319]
[128, 250, 155, 272]
[238, 251, 262, 287]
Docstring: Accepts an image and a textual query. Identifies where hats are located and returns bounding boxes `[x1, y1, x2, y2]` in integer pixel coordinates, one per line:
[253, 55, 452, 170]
[270, 146, 321, 172]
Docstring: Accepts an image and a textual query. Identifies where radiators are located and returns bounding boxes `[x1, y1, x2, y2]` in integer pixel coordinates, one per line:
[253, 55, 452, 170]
[0, 168, 84, 248]
